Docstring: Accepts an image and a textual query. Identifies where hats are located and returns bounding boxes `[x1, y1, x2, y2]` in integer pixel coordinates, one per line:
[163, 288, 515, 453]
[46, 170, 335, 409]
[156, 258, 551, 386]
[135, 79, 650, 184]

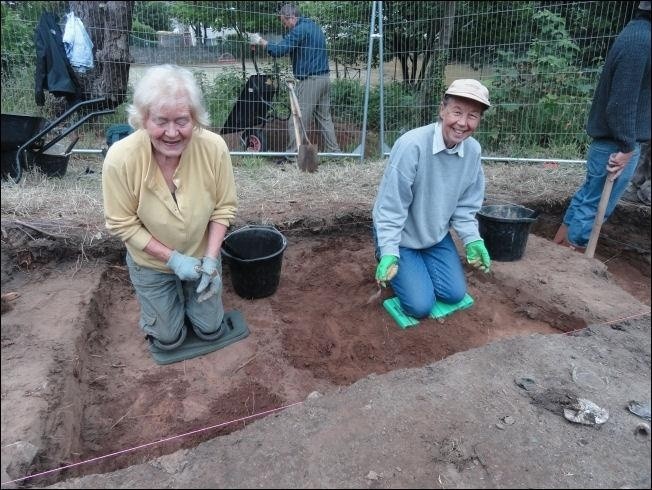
[638, 1, 651, 10]
[445, 79, 492, 107]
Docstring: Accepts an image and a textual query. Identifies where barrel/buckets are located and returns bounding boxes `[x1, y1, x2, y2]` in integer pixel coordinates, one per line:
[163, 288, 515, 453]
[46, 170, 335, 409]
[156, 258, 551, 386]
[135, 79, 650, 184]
[220, 225, 288, 299]
[476, 196, 539, 262]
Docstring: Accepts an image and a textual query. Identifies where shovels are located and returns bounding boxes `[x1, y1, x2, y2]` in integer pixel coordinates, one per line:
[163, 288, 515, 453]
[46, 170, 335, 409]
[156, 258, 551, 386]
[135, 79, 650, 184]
[366, 264, 399, 305]
[284, 78, 318, 174]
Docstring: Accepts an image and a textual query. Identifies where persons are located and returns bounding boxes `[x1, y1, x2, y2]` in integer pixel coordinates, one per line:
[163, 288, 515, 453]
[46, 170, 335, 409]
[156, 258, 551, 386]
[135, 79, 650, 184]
[550, 0, 651, 255]
[250, 3, 342, 172]
[101, 63, 238, 345]
[371, 78, 491, 320]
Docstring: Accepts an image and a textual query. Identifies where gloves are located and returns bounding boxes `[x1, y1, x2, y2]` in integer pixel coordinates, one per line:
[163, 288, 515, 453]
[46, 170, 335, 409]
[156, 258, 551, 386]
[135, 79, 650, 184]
[195, 256, 221, 304]
[375, 256, 399, 288]
[250, 34, 261, 46]
[466, 240, 491, 273]
[166, 249, 201, 280]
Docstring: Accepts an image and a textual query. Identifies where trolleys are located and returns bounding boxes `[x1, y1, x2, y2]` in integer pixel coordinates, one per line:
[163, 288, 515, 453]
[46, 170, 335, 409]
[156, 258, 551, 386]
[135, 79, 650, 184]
[0, 93, 117, 186]
[220, 42, 294, 152]
